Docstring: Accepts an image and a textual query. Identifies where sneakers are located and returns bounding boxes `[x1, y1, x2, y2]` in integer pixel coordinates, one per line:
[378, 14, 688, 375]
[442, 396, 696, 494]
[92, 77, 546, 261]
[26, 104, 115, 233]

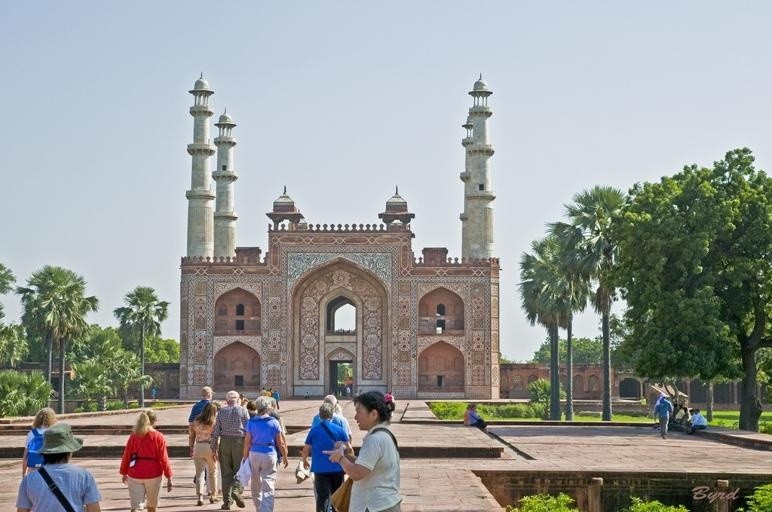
[198, 501, 204, 505]
[221, 504, 230, 510]
[211, 498, 220, 503]
[231, 492, 245, 507]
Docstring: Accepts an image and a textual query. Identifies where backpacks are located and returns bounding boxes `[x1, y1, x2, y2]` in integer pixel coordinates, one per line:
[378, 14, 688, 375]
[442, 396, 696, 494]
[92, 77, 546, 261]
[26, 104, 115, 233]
[27, 429, 44, 468]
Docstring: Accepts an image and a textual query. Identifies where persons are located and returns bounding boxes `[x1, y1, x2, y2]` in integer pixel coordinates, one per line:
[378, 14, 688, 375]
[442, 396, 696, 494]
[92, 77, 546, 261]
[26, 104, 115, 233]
[148, 383, 158, 402]
[653, 392, 708, 440]
[14, 421, 103, 512]
[464, 404, 480, 429]
[469, 404, 489, 434]
[21, 407, 57, 478]
[187, 385, 404, 512]
[118, 408, 173, 512]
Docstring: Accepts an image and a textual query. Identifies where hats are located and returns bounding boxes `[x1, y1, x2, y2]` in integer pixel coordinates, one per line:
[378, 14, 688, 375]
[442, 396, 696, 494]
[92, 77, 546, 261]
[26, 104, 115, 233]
[38, 423, 83, 455]
[661, 393, 667, 397]
[200, 387, 215, 396]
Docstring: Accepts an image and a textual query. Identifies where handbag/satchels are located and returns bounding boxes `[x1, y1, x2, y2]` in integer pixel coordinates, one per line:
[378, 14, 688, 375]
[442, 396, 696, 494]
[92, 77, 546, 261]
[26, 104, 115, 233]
[330, 477, 353, 512]
